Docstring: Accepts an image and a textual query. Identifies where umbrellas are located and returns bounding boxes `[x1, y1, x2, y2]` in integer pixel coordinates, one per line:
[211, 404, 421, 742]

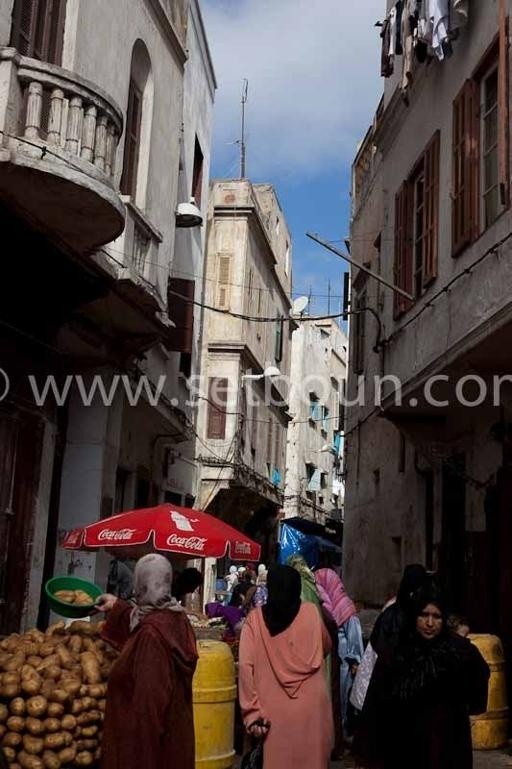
[63, 501, 264, 599]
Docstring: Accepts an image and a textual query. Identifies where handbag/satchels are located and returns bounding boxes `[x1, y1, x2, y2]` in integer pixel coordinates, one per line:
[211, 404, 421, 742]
[242, 738, 262, 769]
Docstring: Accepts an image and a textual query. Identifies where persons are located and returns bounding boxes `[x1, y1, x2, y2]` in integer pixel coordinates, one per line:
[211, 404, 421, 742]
[356, 590, 490, 769]
[315, 567, 365, 762]
[86, 552, 199, 769]
[237, 563, 336, 769]
[201, 561, 270, 661]
[284, 553, 336, 693]
[339, 564, 433, 712]
[173, 568, 205, 603]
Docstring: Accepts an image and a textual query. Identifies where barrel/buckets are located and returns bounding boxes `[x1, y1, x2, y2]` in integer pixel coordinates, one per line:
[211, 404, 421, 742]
[467, 631, 507, 751]
[191, 639, 240, 768]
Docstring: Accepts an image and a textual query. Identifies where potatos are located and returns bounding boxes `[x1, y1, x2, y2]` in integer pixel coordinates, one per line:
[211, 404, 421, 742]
[0, 620, 121, 768]
[53, 590, 92, 606]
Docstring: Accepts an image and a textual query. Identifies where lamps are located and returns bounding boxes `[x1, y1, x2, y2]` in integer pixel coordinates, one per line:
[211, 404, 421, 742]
[241, 367, 282, 386]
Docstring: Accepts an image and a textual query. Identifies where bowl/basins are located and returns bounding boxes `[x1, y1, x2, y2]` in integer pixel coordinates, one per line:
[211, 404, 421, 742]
[45, 575, 103, 618]
